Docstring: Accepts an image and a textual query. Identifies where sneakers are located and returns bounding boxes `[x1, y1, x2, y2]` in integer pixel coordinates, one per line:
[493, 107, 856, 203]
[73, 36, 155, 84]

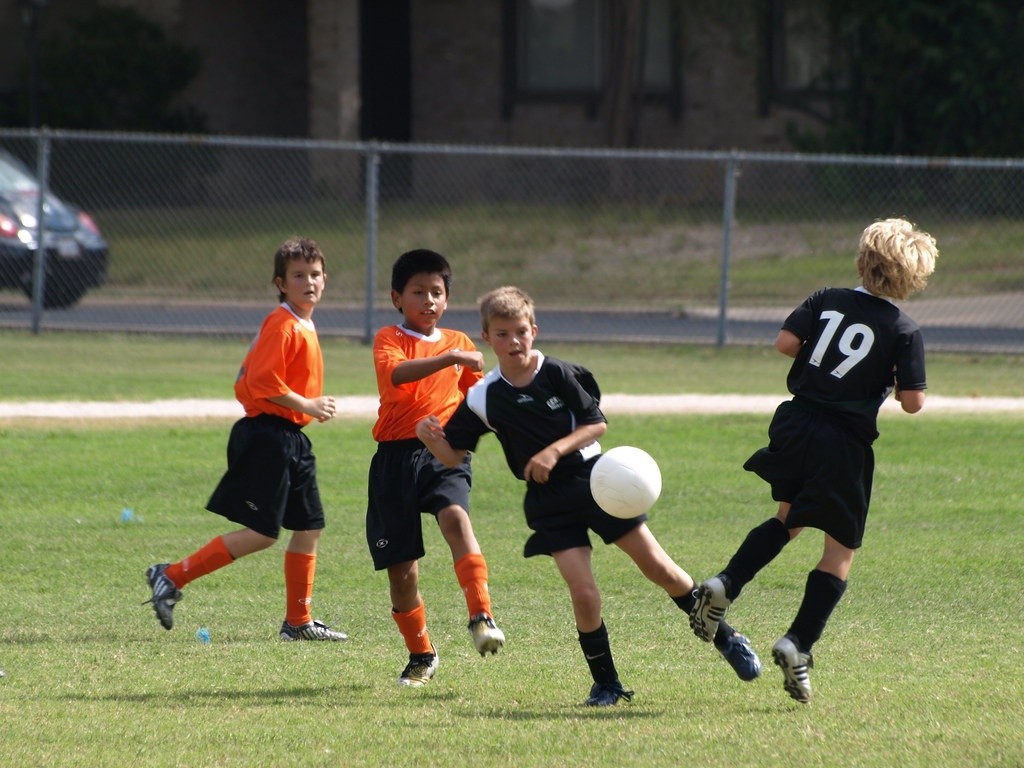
[584, 679, 635, 708]
[714, 631, 762, 682]
[279, 613, 349, 643]
[468, 613, 506, 657]
[771, 633, 814, 704]
[141, 564, 183, 630]
[689, 573, 733, 644]
[397, 642, 439, 688]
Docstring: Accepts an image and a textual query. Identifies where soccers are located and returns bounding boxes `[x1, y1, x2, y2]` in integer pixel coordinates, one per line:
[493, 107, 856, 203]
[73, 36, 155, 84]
[589, 446, 662, 518]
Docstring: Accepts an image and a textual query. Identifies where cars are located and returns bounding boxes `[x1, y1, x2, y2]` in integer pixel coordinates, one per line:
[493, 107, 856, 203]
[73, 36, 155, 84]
[0, 145, 108, 311]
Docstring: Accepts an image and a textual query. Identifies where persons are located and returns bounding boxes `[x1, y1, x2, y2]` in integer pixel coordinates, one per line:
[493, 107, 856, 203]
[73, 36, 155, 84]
[690, 220, 939, 702]
[145, 239, 350, 643]
[366, 250, 505, 689]
[418, 287, 760, 708]
